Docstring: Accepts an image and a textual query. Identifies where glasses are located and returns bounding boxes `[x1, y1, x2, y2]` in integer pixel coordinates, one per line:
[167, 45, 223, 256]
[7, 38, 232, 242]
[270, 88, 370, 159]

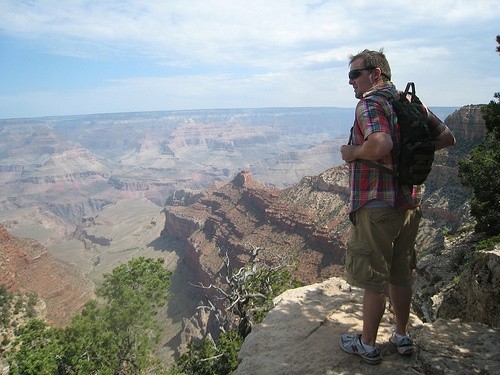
[349, 67, 379, 80]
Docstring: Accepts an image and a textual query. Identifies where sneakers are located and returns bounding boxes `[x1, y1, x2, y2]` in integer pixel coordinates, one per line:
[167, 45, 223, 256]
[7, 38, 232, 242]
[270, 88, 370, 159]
[389, 331, 413, 354]
[339, 334, 381, 365]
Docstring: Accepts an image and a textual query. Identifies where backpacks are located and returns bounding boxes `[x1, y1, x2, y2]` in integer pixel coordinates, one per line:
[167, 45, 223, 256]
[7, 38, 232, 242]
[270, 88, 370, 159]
[370, 83, 434, 187]
